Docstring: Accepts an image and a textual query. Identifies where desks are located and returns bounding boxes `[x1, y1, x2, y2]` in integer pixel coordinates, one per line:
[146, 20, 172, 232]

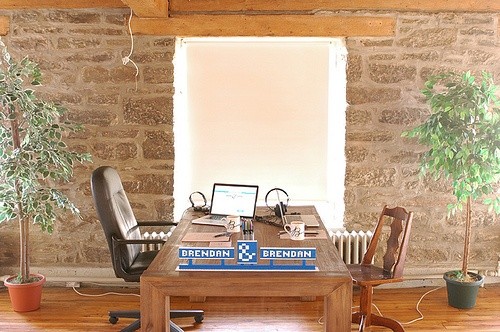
[141, 206, 352, 332]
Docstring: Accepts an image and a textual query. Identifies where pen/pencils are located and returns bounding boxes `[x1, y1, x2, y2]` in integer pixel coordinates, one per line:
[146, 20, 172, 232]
[242, 219, 253, 234]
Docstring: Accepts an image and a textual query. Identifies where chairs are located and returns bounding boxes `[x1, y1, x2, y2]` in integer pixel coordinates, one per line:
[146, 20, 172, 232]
[91, 166, 205, 332]
[345, 205, 413, 332]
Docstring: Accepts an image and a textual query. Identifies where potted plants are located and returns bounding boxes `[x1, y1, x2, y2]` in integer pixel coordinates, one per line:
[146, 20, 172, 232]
[400, 70, 500, 308]
[0, 40, 93, 312]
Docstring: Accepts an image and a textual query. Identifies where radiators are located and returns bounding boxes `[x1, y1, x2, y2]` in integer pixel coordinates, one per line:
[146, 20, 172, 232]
[141, 230, 375, 264]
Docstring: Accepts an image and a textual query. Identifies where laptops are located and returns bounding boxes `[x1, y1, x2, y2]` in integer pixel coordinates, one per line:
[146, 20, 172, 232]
[192, 183, 259, 227]
[276, 191, 319, 228]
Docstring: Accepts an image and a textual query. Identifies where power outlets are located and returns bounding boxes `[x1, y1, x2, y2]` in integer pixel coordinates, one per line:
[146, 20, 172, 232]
[65, 282, 80, 288]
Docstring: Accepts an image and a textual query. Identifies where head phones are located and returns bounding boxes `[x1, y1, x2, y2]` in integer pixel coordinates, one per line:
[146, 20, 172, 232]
[189, 192, 210, 212]
[265, 189, 290, 217]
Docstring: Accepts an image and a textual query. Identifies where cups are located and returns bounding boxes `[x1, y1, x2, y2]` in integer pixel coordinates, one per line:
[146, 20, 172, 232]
[284, 221, 305, 240]
[221, 215, 241, 233]
